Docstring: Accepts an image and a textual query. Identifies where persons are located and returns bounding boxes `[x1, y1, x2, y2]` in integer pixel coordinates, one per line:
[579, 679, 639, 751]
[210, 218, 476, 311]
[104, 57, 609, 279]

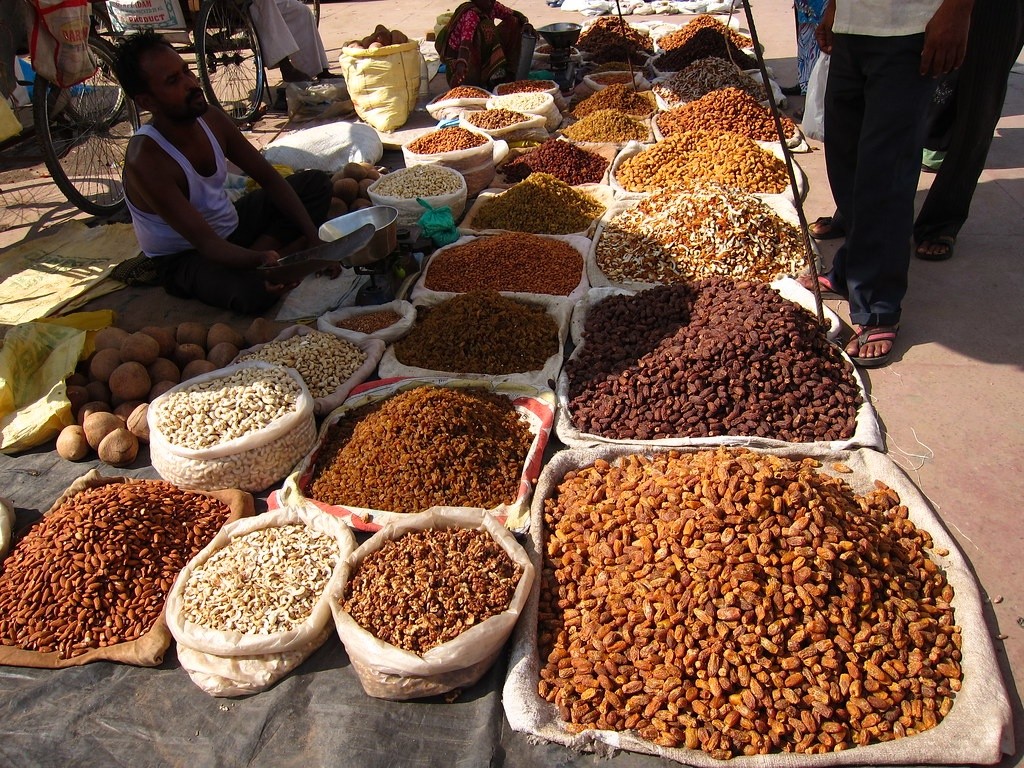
[444, 0, 540, 91]
[250, 0, 344, 82]
[778, 0, 1024, 365]
[113, 28, 342, 317]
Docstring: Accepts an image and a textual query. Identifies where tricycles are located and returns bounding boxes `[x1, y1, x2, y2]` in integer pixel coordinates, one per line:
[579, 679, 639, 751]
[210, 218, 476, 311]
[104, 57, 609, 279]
[17, 0, 265, 217]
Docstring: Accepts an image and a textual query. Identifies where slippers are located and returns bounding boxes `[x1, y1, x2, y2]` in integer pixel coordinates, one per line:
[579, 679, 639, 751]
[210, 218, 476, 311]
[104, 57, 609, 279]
[915, 234, 957, 261]
[843, 324, 900, 366]
[807, 217, 846, 239]
[795, 276, 849, 300]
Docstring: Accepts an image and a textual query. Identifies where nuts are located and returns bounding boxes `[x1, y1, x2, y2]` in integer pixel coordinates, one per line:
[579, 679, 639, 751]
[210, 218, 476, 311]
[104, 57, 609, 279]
[0, 479, 228, 658]
[151, 331, 369, 496]
[335, 522, 523, 697]
[406, 13, 796, 194]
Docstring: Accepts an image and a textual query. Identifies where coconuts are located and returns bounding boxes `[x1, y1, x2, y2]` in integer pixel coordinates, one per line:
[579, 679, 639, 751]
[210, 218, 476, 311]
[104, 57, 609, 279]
[53, 318, 279, 465]
[322, 161, 385, 221]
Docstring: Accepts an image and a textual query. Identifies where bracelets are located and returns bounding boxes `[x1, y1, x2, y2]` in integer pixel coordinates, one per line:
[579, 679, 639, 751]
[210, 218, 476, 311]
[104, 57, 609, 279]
[456, 58, 467, 68]
[523, 23, 529, 25]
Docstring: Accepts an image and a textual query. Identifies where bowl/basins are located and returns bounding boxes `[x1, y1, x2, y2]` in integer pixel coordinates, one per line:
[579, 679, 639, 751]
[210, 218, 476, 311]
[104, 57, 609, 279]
[317, 205, 398, 266]
[536, 23, 582, 48]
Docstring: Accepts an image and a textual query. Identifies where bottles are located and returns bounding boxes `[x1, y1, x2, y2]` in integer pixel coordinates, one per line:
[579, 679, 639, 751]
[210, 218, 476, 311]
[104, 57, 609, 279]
[415, 45, 430, 111]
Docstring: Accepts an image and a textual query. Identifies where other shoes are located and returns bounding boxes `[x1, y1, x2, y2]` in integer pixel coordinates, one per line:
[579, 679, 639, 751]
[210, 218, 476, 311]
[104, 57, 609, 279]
[793, 110, 804, 120]
[781, 84, 802, 95]
[921, 164, 938, 172]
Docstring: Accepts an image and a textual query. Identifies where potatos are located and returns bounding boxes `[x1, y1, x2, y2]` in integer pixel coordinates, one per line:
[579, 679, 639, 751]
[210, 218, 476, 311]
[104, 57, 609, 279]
[345, 24, 407, 52]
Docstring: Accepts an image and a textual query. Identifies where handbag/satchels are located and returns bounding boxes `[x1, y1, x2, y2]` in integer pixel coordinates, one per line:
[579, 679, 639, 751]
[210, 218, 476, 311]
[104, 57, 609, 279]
[802, 51, 831, 142]
[921, 67, 961, 151]
